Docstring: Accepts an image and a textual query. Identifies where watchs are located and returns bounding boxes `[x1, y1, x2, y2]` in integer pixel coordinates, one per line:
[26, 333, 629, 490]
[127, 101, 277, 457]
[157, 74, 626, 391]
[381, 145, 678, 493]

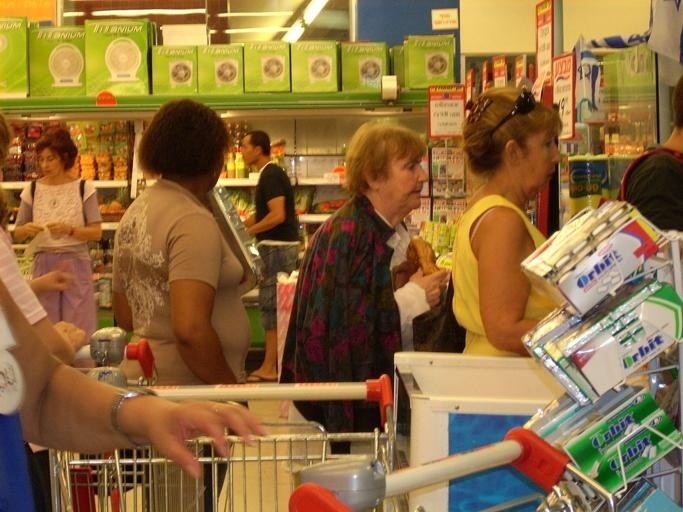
[109, 386, 157, 450]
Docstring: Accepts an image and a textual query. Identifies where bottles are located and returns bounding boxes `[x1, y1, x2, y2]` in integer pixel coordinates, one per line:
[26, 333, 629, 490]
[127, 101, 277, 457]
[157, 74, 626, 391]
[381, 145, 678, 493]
[226, 151, 235, 179]
[234, 153, 244, 178]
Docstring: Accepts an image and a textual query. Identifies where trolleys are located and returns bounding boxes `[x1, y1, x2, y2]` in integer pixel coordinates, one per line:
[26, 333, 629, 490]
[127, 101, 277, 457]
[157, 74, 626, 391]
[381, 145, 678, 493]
[50, 374, 399, 512]
[288, 426, 618, 512]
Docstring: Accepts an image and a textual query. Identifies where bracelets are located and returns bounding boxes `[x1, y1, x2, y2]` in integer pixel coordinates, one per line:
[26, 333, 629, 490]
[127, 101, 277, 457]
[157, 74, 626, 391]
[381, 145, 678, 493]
[68, 225, 76, 236]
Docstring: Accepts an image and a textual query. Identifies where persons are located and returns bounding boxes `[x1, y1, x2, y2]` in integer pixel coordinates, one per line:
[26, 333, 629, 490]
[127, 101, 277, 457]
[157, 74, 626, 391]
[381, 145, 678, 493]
[111, 100, 255, 511]
[451, 88, 563, 357]
[241, 131, 302, 383]
[278, 121, 449, 455]
[31, 271, 77, 294]
[618, 76, 683, 232]
[0, 280, 268, 512]
[0, 111, 87, 365]
[14, 129, 102, 368]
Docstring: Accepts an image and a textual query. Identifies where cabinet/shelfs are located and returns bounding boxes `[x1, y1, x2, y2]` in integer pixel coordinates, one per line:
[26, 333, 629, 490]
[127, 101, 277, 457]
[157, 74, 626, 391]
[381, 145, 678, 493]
[0, 87, 436, 351]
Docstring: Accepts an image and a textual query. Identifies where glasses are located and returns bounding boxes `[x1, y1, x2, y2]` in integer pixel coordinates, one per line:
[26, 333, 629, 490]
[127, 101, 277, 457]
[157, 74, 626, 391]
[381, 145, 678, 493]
[487, 88, 537, 137]
